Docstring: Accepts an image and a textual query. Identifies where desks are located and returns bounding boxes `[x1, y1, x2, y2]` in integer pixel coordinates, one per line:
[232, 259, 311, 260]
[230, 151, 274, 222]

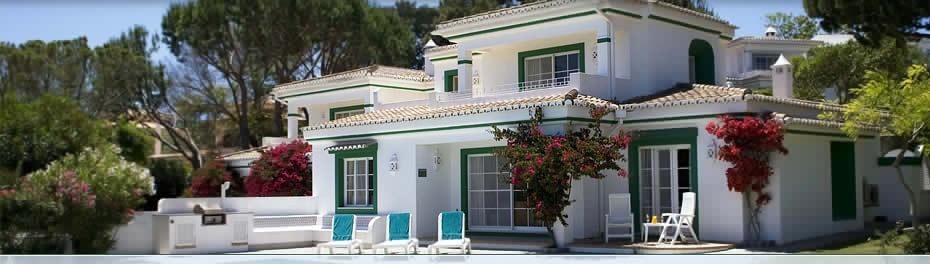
[640, 222, 671, 243]
[152, 212, 250, 254]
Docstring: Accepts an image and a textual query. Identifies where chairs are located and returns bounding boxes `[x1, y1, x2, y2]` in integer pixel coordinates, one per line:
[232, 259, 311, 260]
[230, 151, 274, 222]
[372, 213, 419, 255]
[427, 211, 471, 255]
[605, 193, 635, 243]
[317, 215, 362, 255]
[658, 192, 700, 244]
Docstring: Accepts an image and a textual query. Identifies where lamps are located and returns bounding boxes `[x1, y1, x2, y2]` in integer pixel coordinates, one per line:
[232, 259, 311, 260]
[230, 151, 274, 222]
[592, 46, 600, 65]
[434, 152, 442, 170]
[472, 73, 481, 89]
[390, 155, 399, 174]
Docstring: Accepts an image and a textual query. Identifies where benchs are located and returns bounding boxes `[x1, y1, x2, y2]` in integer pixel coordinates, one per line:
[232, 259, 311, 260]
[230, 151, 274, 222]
[251, 214, 384, 248]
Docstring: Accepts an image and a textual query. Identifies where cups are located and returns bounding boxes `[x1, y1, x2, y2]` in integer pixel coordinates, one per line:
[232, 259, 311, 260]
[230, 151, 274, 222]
[651, 216, 657, 224]
